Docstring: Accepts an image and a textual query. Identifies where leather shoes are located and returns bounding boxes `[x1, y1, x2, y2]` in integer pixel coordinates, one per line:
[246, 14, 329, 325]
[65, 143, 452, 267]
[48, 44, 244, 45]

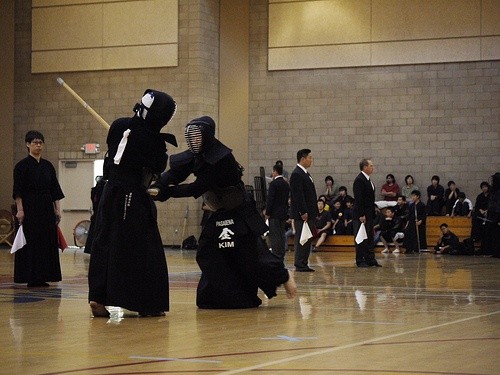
[356, 260, 370, 268]
[365, 258, 383, 268]
[295, 267, 316, 273]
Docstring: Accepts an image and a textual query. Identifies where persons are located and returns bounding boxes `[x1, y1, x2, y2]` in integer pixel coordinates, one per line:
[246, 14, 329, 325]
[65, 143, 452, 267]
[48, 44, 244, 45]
[319, 175, 354, 235]
[444, 180, 461, 216]
[263, 165, 290, 259]
[289, 148, 317, 272]
[343, 198, 354, 235]
[402, 190, 427, 253]
[352, 157, 383, 268]
[450, 192, 474, 218]
[13, 129, 65, 288]
[312, 199, 332, 252]
[471, 181, 490, 243]
[379, 206, 405, 253]
[433, 223, 461, 256]
[271, 160, 289, 181]
[373, 204, 385, 248]
[425, 175, 445, 216]
[146, 119, 298, 309]
[83, 88, 179, 318]
[381, 173, 400, 201]
[394, 196, 410, 229]
[474, 173, 500, 259]
[401, 174, 419, 206]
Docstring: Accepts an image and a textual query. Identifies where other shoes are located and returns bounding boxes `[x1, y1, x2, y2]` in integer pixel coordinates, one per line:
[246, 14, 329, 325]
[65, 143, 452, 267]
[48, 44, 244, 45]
[312, 246, 318, 253]
[393, 248, 401, 253]
[381, 249, 391, 253]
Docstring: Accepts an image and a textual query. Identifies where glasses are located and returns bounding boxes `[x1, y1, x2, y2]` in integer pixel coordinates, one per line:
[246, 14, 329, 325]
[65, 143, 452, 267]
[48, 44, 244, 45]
[31, 141, 44, 146]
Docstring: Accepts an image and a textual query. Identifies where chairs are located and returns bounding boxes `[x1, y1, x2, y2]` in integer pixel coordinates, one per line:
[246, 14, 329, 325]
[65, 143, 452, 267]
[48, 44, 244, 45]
[0, 209, 14, 249]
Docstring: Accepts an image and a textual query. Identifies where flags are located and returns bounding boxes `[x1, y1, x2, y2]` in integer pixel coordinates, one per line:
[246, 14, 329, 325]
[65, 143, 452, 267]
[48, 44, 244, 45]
[55, 224, 68, 252]
[354, 222, 369, 244]
[299, 220, 312, 246]
[10, 225, 26, 255]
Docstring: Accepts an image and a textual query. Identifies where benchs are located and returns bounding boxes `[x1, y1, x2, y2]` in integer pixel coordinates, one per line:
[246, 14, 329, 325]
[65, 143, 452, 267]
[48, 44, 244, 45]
[281, 234, 408, 253]
[426, 216, 472, 247]
